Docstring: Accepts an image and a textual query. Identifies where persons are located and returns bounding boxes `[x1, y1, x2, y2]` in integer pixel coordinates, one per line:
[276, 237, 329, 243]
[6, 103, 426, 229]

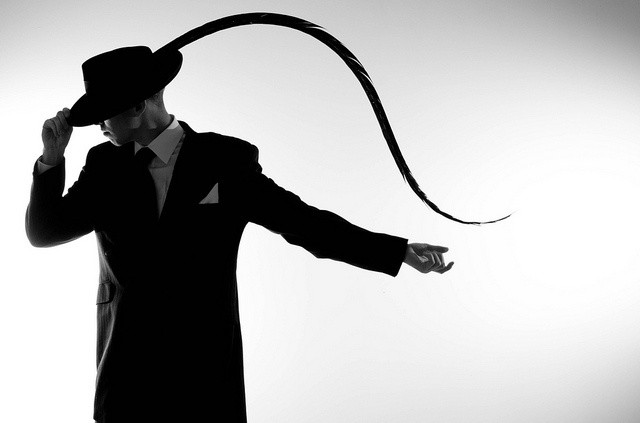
[24, 46, 455, 423]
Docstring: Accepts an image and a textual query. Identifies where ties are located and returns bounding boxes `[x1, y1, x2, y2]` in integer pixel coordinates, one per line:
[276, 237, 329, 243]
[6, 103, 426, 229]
[134, 147, 159, 226]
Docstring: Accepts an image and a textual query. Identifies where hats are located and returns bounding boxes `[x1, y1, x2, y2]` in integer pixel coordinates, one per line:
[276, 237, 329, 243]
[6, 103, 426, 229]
[65, 45, 183, 127]
